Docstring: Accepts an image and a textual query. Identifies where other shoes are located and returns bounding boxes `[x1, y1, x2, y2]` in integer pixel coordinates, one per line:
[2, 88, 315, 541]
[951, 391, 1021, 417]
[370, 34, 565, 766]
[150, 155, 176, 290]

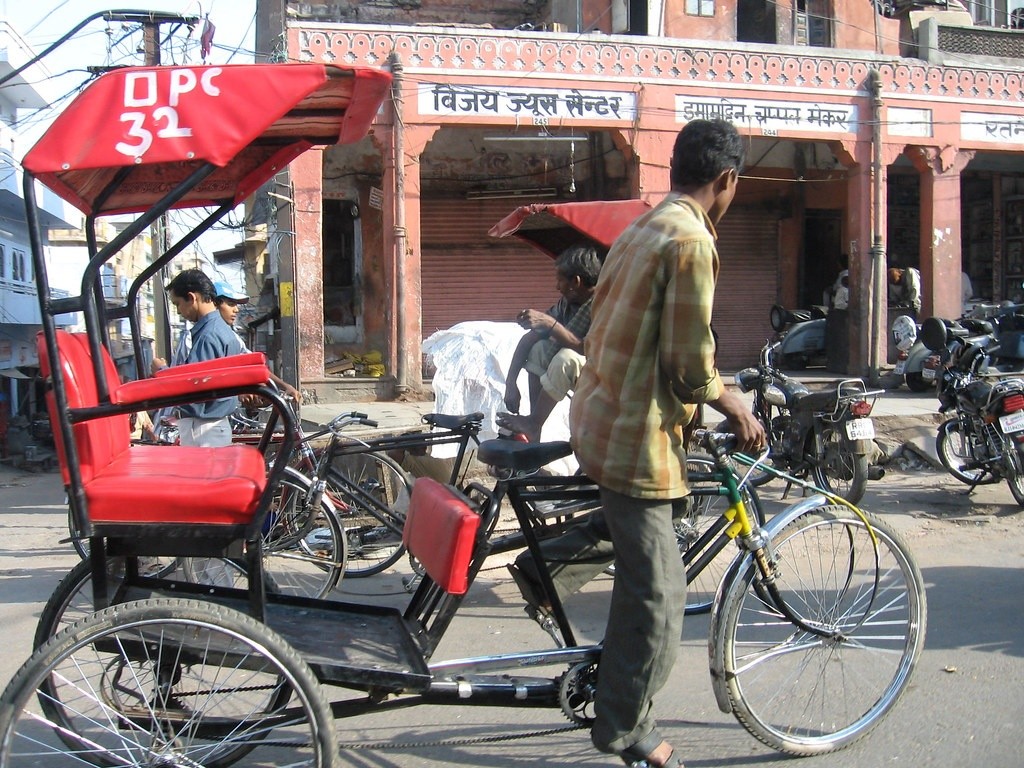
[108, 553, 166, 574]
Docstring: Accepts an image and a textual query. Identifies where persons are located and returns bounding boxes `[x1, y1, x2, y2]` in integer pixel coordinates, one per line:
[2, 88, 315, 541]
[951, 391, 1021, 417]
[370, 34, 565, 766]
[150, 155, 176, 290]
[888, 266, 921, 313]
[506, 117, 766, 768]
[961, 272, 973, 318]
[150, 269, 242, 587]
[830, 254, 849, 311]
[153, 281, 302, 444]
[495, 241, 606, 442]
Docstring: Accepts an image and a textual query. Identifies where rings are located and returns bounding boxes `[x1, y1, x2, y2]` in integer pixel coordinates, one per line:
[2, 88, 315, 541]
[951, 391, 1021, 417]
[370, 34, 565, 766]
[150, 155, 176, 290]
[754, 442, 761, 447]
[522, 310, 526, 314]
[521, 315, 524, 320]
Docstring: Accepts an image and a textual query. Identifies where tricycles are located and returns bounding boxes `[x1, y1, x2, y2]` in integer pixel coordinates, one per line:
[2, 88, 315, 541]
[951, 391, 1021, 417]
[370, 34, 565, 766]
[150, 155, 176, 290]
[8, 57, 930, 765]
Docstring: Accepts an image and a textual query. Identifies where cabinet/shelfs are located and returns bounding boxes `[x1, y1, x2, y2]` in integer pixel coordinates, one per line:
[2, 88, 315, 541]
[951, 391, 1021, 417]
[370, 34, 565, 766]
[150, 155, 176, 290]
[962, 192, 1024, 359]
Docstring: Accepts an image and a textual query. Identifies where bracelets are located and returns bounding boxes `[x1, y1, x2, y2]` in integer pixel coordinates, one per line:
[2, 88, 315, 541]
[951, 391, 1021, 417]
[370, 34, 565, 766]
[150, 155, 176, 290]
[547, 320, 558, 333]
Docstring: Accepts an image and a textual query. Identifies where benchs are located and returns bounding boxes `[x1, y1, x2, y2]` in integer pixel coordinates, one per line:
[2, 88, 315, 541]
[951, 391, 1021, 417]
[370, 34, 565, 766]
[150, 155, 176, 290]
[35, 327, 297, 628]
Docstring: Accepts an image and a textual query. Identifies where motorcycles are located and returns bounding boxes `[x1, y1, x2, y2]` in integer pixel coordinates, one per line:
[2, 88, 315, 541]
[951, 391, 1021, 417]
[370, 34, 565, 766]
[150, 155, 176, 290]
[934, 335, 1024, 505]
[711, 339, 890, 505]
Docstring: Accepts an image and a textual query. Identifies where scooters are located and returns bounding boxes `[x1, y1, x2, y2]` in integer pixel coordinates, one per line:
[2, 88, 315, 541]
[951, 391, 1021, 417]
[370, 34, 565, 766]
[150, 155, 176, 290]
[770, 268, 1023, 394]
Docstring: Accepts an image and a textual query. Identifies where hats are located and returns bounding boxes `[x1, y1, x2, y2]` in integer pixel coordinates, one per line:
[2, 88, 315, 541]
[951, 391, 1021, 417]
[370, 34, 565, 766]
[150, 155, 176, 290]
[212, 281, 250, 304]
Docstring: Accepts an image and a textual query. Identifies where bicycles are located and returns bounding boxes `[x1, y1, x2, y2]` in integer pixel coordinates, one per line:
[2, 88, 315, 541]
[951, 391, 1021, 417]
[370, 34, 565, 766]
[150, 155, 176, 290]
[113, 358, 519, 580]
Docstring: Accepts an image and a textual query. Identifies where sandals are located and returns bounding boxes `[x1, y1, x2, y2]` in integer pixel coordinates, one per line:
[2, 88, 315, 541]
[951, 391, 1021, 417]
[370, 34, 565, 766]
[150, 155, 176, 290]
[591, 722, 685, 768]
[506, 559, 559, 630]
